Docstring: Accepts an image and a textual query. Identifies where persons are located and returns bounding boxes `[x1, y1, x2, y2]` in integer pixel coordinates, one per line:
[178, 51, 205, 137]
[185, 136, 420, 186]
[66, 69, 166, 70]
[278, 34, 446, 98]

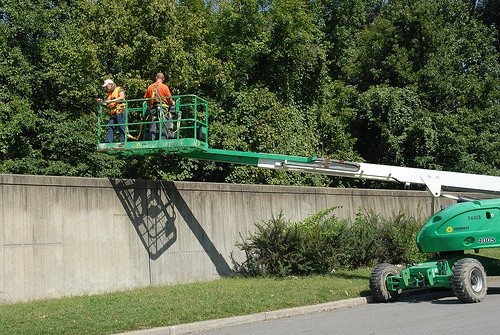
[144, 73, 175, 141]
[97, 79, 127, 143]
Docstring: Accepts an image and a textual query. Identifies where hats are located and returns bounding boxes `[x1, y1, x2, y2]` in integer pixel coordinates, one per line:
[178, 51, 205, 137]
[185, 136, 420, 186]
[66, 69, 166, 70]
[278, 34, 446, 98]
[102, 79, 114, 88]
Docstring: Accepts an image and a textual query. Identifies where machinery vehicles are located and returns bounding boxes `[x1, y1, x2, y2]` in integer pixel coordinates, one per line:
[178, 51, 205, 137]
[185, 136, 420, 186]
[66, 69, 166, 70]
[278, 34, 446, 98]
[96, 93, 499, 303]
